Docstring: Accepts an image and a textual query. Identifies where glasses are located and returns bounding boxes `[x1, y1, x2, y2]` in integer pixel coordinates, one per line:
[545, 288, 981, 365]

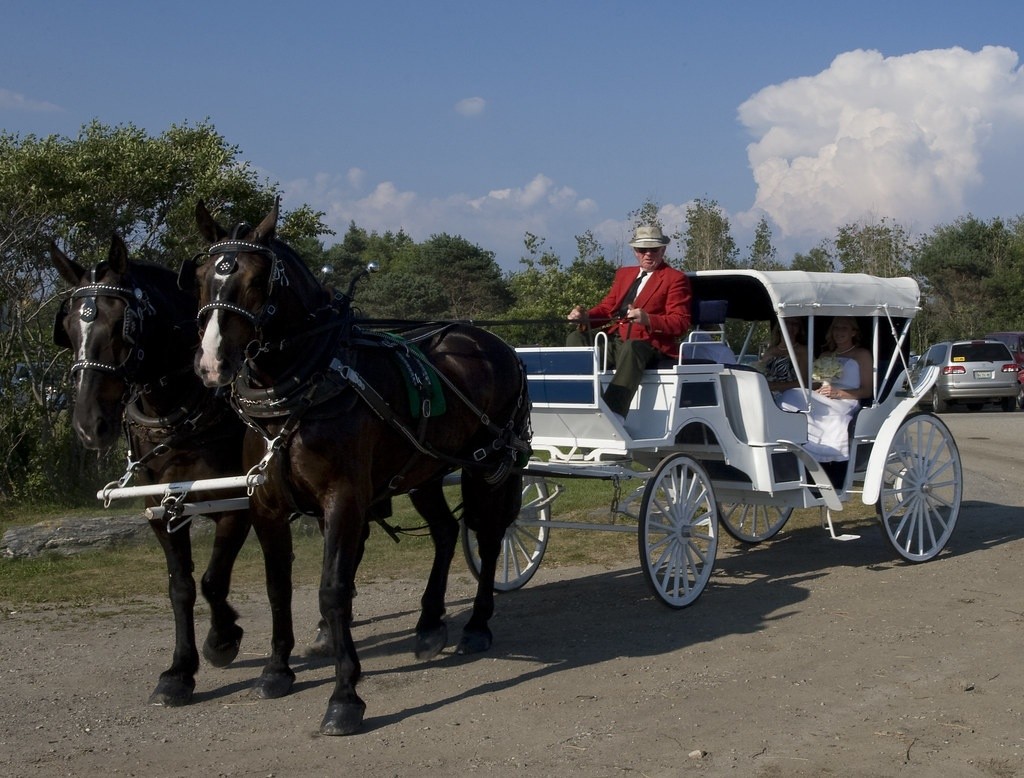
[634, 247, 660, 254]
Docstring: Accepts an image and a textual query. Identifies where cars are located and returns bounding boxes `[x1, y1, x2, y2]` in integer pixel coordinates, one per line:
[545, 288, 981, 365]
[1017, 370, 1024, 411]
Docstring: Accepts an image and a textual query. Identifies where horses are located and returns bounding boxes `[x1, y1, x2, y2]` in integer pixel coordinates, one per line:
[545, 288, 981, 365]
[47, 195, 529, 738]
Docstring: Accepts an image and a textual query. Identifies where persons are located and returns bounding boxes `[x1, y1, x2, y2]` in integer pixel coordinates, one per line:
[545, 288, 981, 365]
[566, 227, 690, 418]
[777, 317, 873, 463]
[751, 319, 808, 401]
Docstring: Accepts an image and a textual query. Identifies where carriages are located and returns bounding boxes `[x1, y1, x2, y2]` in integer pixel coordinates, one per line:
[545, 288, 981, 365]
[45, 189, 965, 737]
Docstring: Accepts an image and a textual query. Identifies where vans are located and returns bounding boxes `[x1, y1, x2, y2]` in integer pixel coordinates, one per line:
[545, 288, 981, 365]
[984, 331, 1024, 368]
[909, 338, 1019, 414]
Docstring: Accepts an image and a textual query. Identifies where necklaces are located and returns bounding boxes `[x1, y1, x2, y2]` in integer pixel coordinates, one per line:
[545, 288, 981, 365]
[835, 346, 855, 354]
[778, 343, 798, 351]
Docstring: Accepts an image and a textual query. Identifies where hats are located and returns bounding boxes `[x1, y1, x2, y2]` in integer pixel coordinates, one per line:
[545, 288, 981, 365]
[628, 226, 670, 247]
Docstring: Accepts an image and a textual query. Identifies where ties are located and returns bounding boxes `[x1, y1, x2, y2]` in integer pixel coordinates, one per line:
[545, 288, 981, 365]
[616, 270, 647, 320]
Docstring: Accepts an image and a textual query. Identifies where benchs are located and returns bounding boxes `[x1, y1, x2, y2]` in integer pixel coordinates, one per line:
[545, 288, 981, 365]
[601, 300, 811, 447]
[849, 357, 914, 439]
